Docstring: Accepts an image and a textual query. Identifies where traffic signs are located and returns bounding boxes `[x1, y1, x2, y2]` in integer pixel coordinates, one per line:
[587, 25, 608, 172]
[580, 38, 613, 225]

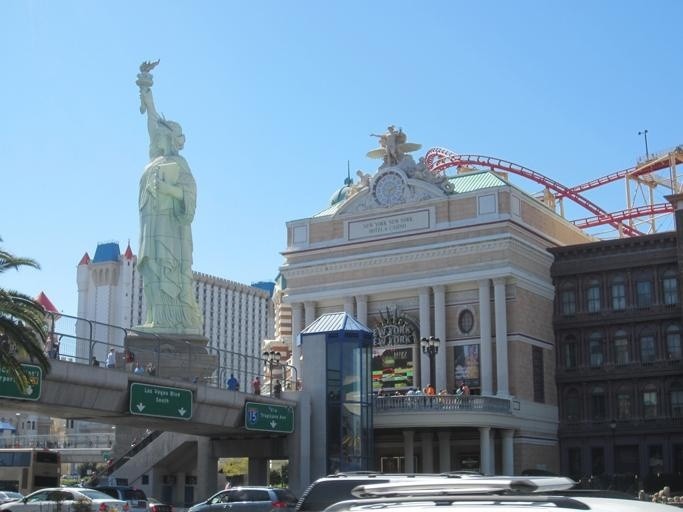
[0, 361, 41, 401]
[244, 401, 293, 435]
[128, 381, 192, 422]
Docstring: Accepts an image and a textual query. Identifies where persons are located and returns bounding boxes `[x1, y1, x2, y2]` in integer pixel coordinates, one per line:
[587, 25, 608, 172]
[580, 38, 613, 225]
[129, 58, 206, 335]
[296, 377, 302, 392]
[226, 372, 239, 391]
[224, 475, 233, 502]
[78, 478, 85, 487]
[344, 170, 371, 200]
[130, 438, 137, 455]
[268, 479, 274, 487]
[146, 428, 152, 442]
[412, 156, 439, 185]
[368, 123, 400, 168]
[372, 379, 471, 410]
[272, 378, 282, 400]
[253, 376, 261, 395]
[106, 457, 113, 473]
[286, 381, 291, 391]
[90, 470, 99, 486]
[90, 344, 155, 377]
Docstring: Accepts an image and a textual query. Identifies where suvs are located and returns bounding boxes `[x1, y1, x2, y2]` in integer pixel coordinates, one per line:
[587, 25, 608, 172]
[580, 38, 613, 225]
[290, 472, 503, 512]
[183, 484, 305, 512]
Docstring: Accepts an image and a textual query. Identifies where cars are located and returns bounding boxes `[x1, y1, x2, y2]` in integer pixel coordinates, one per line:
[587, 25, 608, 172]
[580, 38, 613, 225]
[319, 472, 681, 512]
[0, 447, 170, 512]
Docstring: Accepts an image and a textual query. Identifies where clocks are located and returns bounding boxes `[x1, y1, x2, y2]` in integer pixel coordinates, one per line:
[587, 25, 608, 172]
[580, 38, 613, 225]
[373, 171, 404, 208]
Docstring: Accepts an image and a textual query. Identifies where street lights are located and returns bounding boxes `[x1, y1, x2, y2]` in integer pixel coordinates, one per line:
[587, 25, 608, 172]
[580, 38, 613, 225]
[419, 335, 440, 390]
[262, 351, 281, 397]
